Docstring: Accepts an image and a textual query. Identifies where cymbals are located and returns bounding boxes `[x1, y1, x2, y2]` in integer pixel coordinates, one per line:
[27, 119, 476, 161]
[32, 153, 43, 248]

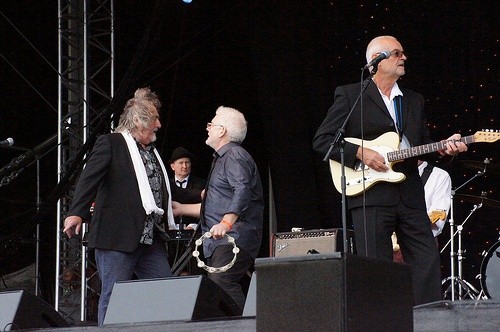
[192, 230, 239, 274]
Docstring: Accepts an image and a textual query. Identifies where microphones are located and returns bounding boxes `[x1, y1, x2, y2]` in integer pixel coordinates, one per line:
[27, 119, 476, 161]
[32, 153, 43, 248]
[0, 137, 14, 148]
[365, 49, 390, 68]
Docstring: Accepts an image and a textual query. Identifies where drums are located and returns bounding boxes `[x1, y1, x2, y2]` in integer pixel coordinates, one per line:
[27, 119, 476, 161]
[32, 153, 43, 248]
[480, 240, 500, 302]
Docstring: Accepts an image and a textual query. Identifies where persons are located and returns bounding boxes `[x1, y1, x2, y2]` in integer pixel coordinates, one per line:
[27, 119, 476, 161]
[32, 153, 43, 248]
[167, 147, 203, 230]
[171, 106, 264, 321]
[63, 87, 207, 326]
[312, 36, 467, 305]
[418, 157, 452, 250]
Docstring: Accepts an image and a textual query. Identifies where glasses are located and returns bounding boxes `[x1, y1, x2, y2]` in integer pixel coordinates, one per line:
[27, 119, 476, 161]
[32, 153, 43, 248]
[207, 123, 227, 132]
[389, 51, 403, 58]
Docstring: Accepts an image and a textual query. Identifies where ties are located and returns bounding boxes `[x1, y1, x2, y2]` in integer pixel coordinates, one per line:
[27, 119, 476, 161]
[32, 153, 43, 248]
[176, 180, 187, 190]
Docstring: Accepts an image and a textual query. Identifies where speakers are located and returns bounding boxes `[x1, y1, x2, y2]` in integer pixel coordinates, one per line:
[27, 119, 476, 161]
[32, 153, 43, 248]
[274, 229, 357, 257]
[102, 275, 243, 327]
[0, 289, 70, 332]
[254, 251, 414, 332]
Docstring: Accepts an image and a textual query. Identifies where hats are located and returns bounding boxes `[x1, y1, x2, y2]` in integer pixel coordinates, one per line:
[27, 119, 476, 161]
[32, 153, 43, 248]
[166, 148, 192, 164]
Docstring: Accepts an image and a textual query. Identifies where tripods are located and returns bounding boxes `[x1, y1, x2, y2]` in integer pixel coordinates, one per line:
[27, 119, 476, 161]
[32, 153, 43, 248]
[438, 162, 487, 301]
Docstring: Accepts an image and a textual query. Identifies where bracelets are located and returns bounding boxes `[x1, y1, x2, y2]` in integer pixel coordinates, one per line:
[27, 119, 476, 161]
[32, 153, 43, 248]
[220, 221, 233, 231]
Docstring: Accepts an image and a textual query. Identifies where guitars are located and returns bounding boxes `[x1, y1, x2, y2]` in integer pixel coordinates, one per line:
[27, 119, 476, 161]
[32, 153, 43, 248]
[391, 209, 448, 251]
[328, 128, 500, 197]
